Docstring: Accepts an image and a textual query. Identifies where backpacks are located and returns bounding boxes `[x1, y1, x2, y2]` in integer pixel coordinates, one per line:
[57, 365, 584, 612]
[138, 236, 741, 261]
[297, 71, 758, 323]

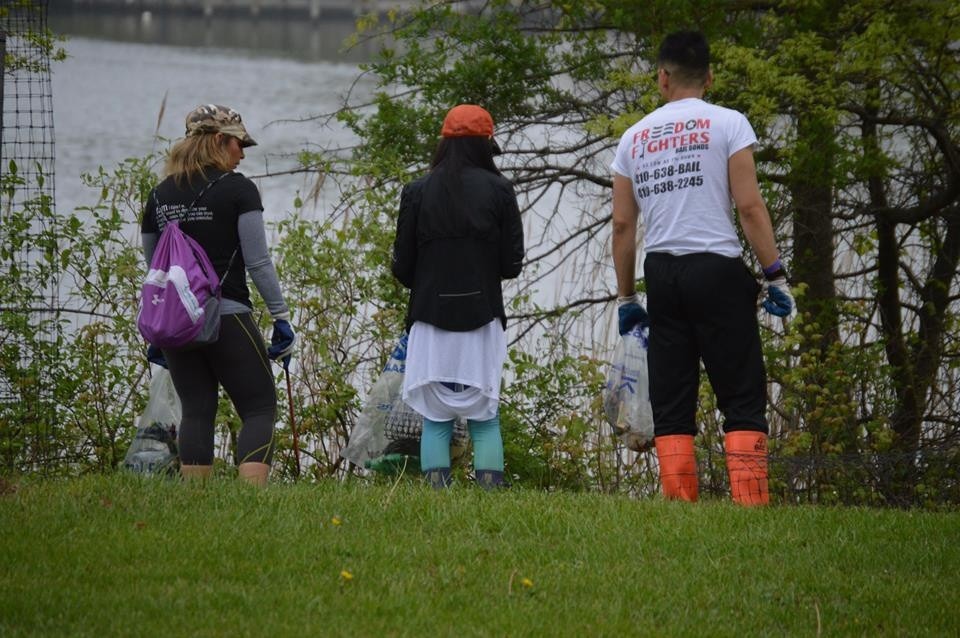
[134, 172, 246, 354]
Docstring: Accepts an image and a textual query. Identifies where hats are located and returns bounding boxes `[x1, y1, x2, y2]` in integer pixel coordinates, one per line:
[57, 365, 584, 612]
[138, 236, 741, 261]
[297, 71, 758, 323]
[440, 105, 503, 156]
[184, 103, 258, 148]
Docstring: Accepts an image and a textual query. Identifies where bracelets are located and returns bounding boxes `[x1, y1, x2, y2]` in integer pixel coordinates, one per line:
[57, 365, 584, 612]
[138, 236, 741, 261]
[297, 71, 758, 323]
[764, 259, 782, 274]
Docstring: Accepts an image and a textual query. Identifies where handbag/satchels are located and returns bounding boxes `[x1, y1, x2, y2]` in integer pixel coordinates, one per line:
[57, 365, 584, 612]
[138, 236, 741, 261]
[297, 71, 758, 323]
[382, 367, 470, 449]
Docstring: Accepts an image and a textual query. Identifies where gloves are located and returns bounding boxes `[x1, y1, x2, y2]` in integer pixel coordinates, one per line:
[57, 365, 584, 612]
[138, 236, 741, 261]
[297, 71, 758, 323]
[615, 293, 648, 347]
[762, 275, 793, 318]
[144, 345, 168, 370]
[266, 319, 295, 368]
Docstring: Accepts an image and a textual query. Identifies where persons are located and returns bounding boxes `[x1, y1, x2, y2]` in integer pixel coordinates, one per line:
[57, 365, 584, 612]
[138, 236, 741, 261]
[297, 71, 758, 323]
[141, 105, 297, 485]
[391, 103, 525, 488]
[611, 28, 798, 509]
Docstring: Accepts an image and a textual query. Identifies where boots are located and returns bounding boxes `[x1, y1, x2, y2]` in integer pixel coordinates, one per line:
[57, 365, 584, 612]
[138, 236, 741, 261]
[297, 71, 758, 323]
[472, 469, 504, 493]
[724, 430, 770, 506]
[422, 466, 450, 491]
[653, 435, 699, 504]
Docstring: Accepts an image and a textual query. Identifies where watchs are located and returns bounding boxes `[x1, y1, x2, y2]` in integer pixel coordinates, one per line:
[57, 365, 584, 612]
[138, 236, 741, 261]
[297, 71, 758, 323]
[766, 265, 786, 280]
